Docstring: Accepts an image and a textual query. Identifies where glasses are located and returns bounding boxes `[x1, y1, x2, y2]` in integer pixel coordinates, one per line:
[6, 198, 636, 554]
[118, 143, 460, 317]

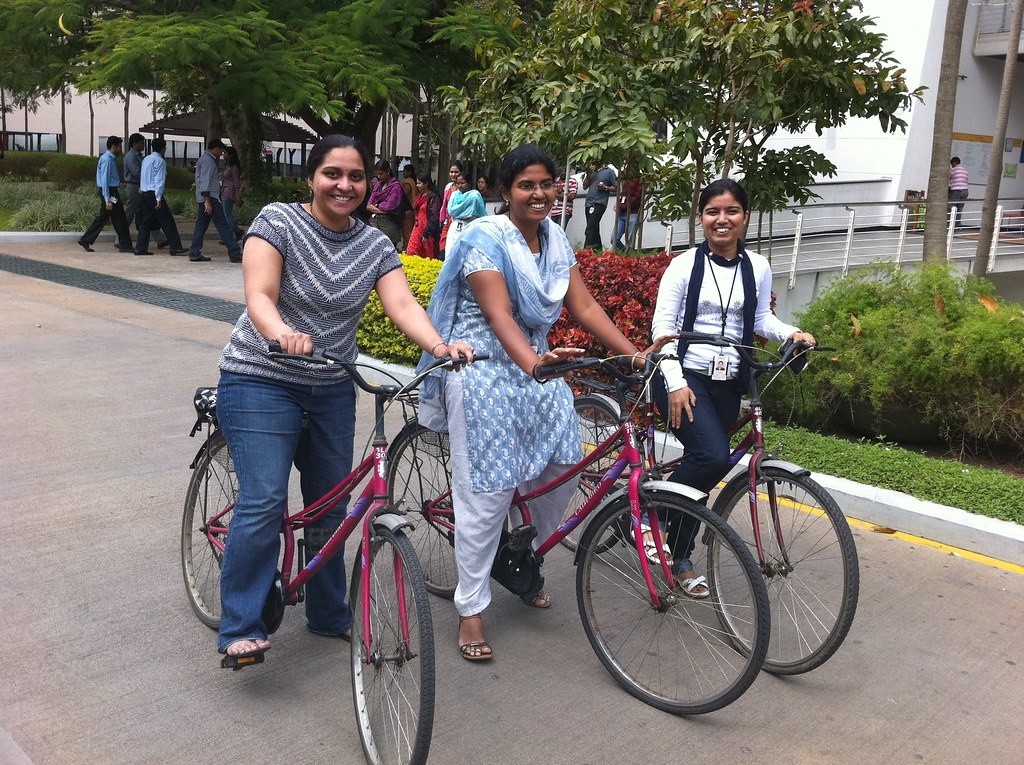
[506, 180, 558, 192]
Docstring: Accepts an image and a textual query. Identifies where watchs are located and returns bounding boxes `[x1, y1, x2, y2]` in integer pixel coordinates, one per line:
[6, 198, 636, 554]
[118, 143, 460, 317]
[375, 203, 379, 208]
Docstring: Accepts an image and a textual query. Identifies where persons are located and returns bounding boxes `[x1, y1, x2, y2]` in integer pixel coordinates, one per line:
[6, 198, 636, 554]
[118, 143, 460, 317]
[133, 138, 189, 255]
[445, 172, 486, 262]
[476, 175, 496, 198]
[401, 164, 420, 254]
[611, 158, 648, 255]
[114, 133, 170, 249]
[365, 159, 403, 254]
[583, 160, 619, 255]
[188, 137, 243, 263]
[77, 135, 135, 252]
[629, 178, 817, 595]
[216, 134, 473, 656]
[442, 143, 680, 659]
[219, 146, 245, 245]
[947, 157, 968, 232]
[405, 173, 442, 260]
[438, 160, 464, 264]
[551, 167, 578, 232]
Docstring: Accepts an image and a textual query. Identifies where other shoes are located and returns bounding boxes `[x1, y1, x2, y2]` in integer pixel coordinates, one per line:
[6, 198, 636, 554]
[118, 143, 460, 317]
[119, 247, 135, 252]
[134, 250, 153, 255]
[77, 240, 95, 252]
[190, 256, 211, 261]
[218, 241, 226, 246]
[158, 240, 169, 249]
[171, 247, 189, 255]
[235, 229, 245, 241]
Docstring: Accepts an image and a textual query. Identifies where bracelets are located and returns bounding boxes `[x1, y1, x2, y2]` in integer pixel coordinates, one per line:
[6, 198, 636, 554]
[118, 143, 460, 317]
[630, 351, 642, 372]
[432, 342, 448, 359]
[533, 362, 549, 384]
[605, 185, 607, 191]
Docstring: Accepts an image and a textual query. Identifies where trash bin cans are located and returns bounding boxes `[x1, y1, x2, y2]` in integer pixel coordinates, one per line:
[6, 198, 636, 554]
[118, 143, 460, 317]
[905, 190, 925, 230]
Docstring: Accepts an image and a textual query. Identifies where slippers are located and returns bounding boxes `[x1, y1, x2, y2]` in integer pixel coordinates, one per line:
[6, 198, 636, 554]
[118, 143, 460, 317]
[226, 639, 272, 658]
[341, 629, 373, 644]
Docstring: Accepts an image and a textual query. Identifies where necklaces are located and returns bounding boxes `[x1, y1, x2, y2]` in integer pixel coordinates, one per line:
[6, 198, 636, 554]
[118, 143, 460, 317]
[508, 209, 543, 259]
[310, 202, 350, 230]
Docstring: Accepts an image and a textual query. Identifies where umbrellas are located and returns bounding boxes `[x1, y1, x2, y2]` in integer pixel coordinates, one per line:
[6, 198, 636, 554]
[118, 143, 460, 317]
[138, 109, 319, 144]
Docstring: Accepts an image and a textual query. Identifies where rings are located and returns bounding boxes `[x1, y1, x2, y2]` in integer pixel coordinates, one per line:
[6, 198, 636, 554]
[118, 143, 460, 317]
[467, 345, 472, 351]
[811, 338, 815, 340]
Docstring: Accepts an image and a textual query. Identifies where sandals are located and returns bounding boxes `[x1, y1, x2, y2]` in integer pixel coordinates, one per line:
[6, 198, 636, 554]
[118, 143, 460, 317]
[670, 570, 710, 599]
[631, 524, 674, 565]
[521, 589, 551, 607]
[458, 612, 493, 660]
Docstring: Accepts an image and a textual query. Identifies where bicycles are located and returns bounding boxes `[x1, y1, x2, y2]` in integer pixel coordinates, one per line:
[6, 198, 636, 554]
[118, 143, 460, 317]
[557, 332, 862, 679]
[180, 341, 490, 765]
[384, 333, 772, 718]
